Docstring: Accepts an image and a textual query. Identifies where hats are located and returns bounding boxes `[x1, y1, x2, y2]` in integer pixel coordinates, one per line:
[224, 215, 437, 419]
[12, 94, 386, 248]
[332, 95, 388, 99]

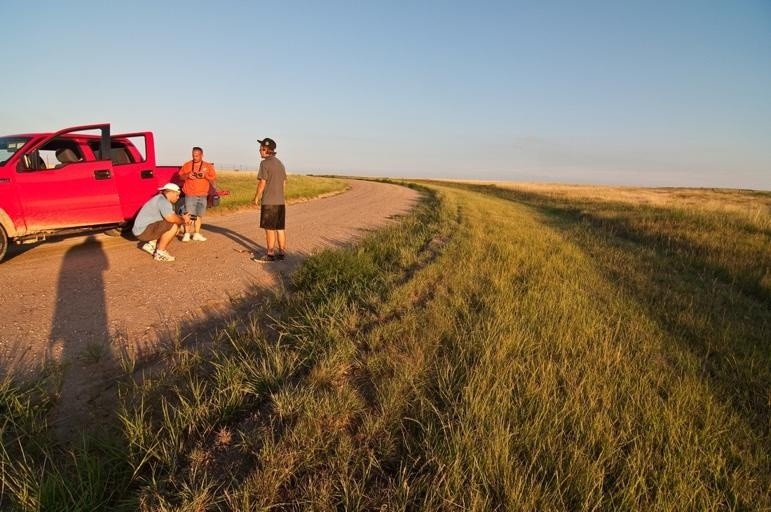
[256, 137, 277, 151]
[158, 183, 180, 193]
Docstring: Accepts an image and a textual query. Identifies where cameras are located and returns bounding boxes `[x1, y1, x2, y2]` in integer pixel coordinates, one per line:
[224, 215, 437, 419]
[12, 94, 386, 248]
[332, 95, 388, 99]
[182, 213, 198, 220]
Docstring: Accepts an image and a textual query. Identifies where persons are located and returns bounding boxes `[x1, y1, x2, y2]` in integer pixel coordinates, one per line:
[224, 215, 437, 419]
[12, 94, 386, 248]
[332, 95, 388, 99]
[252, 138, 289, 262]
[178, 147, 218, 241]
[130, 183, 194, 262]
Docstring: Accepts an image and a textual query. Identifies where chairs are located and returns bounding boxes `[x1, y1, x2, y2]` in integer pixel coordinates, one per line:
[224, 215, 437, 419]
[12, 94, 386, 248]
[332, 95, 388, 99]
[53, 146, 79, 168]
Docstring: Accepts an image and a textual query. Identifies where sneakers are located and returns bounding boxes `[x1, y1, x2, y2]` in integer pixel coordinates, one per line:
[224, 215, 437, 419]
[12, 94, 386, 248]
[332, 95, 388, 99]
[182, 233, 191, 242]
[276, 254, 285, 262]
[154, 248, 175, 262]
[254, 253, 276, 263]
[143, 242, 157, 254]
[192, 233, 206, 242]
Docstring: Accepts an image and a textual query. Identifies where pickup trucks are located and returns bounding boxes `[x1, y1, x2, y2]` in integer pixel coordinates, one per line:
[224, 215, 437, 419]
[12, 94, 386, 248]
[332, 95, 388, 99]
[0, 118, 231, 264]
